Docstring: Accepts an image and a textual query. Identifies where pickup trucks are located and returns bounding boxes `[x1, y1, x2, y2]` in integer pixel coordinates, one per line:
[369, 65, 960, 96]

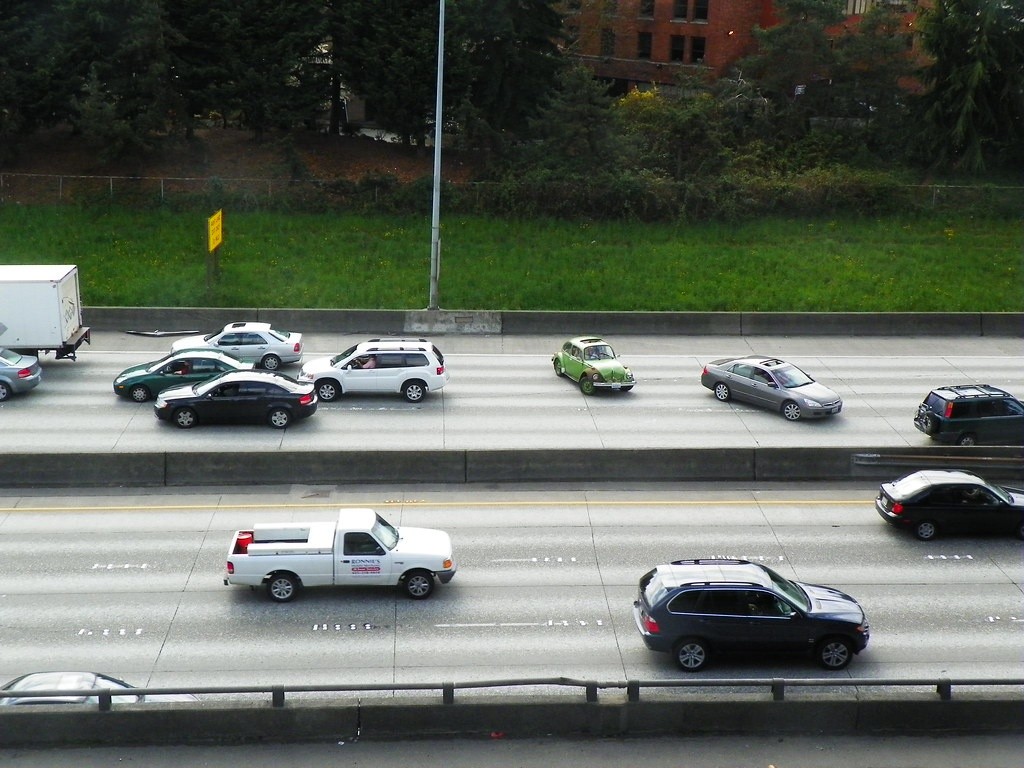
[223, 507, 458, 602]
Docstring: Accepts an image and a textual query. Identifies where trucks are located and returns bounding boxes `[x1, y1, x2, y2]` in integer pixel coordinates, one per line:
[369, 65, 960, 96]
[0, 264, 91, 362]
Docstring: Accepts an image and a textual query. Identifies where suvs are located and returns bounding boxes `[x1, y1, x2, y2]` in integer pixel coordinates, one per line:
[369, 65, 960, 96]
[914, 384, 1024, 446]
[633, 558, 870, 672]
[296, 338, 451, 403]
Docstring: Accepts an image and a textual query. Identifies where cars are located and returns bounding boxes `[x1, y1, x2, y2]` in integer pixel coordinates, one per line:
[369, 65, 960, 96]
[0, 671, 200, 705]
[551, 336, 636, 396]
[0, 347, 43, 402]
[170, 322, 304, 371]
[875, 469, 1024, 540]
[701, 355, 842, 421]
[113, 348, 256, 403]
[154, 369, 319, 429]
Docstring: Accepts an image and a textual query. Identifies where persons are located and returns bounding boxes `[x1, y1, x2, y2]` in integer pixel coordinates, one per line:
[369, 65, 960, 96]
[356, 354, 375, 369]
[587, 348, 599, 358]
[958, 484, 980, 501]
[173, 360, 192, 375]
